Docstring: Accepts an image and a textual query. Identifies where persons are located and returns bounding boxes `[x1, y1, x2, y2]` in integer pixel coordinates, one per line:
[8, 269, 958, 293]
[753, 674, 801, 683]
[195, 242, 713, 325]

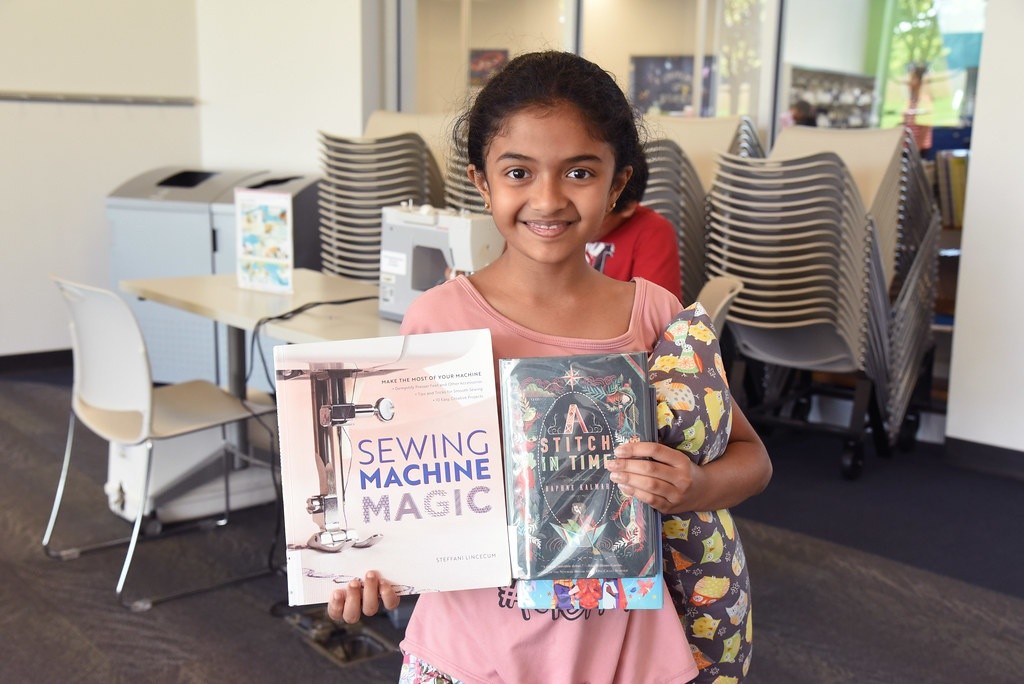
[781, 101, 809, 132]
[554, 579, 580, 608]
[584, 145, 682, 302]
[326, 51, 771, 684]
[602, 578, 626, 609]
[574, 578, 602, 608]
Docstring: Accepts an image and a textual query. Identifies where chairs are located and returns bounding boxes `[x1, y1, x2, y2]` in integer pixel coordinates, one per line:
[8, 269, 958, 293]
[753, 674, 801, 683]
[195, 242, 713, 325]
[316, 112, 944, 476]
[42, 275, 287, 611]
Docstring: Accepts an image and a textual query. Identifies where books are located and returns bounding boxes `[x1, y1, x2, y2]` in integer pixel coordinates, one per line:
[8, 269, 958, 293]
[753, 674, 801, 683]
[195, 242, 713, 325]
[271, 329, 512, 605]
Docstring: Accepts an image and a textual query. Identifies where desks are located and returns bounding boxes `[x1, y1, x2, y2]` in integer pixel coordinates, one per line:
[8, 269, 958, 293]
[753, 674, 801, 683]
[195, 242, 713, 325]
[122, 269, 402, 469]
